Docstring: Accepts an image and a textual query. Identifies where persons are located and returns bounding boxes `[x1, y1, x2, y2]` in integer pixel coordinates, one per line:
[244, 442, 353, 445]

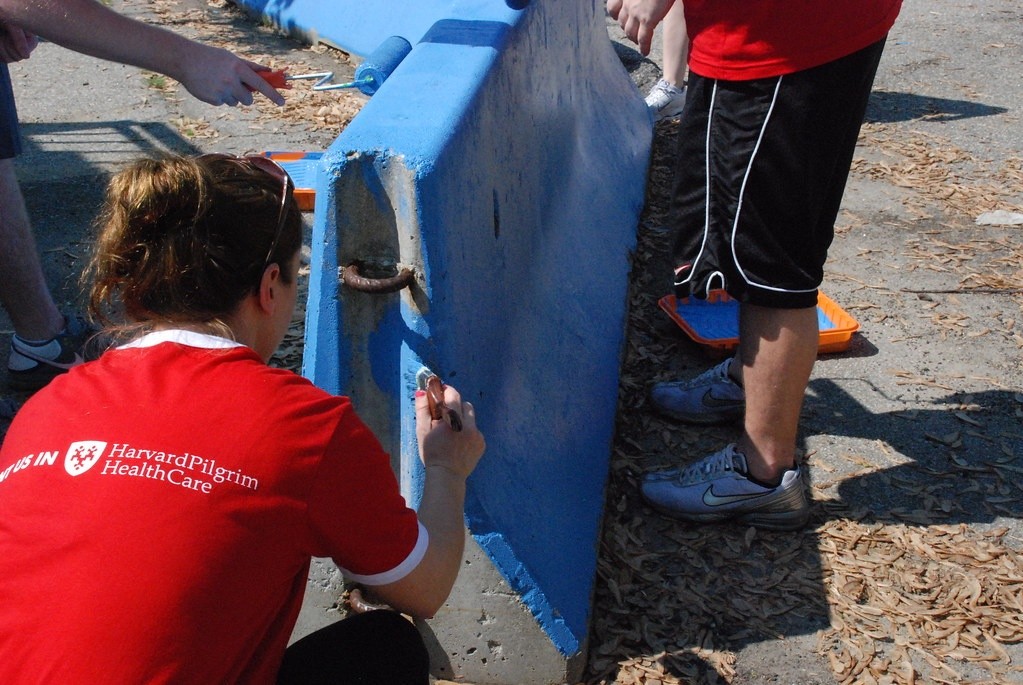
[0, 154, 489, 685]
[0, 1, 287, 383]
[642, 1, 691, 122]
[603, 0, 904, 533]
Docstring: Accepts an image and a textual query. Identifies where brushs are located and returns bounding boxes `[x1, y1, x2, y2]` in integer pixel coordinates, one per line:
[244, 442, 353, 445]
[415, 366, 467, 434]
[247, 35, 416, 95]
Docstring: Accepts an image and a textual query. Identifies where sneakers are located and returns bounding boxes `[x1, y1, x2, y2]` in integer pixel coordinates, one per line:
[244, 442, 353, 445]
[6, 314, 104, 392]
[639, 442, 811, 530]
[648, 357, 747, 423]
